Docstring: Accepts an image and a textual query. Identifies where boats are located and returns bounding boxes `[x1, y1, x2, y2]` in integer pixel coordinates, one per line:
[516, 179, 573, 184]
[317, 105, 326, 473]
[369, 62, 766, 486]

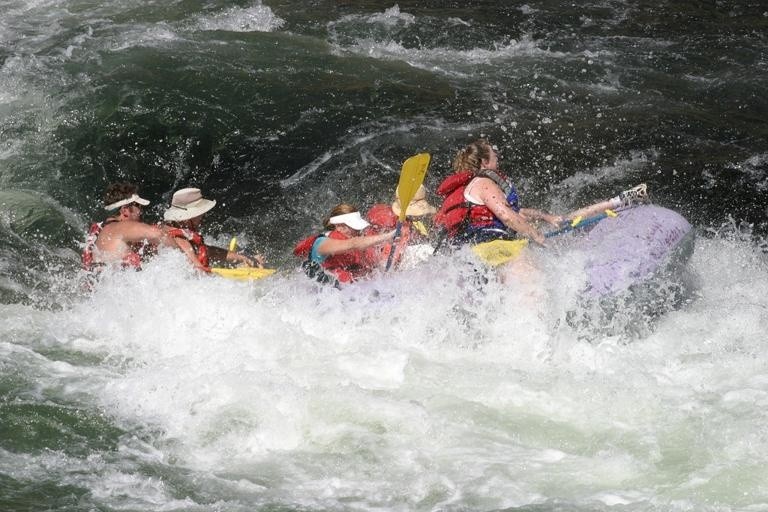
[580, 205, 696, 322]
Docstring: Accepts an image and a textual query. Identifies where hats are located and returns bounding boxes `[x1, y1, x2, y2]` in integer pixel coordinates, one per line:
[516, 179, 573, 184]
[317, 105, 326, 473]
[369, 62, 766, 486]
[162, 187, 218, 222]
[104, 193, 151, 211]
[329, 210, 370, 231]
[391, 183, 438, 217]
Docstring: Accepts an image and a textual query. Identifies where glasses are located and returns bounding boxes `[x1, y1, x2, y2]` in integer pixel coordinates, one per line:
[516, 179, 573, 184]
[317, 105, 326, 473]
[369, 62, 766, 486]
[130, 201, 144, 211]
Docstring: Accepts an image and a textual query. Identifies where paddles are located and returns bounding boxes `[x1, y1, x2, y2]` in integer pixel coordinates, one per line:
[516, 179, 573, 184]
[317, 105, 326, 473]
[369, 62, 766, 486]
[472, 210, 619, 264]
[193, 267, 276, 280]
[384, 153, 430, 273]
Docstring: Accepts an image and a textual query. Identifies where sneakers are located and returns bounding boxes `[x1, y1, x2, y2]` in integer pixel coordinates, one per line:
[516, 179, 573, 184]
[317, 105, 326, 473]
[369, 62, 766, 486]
[618, 181, 650, 206]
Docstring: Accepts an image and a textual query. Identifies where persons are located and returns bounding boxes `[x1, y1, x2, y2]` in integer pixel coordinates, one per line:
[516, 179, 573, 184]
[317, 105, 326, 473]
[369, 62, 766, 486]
[291, 183, 438, 292]
[434, 140, 563, 277]
[83, 182, 265, 275]
